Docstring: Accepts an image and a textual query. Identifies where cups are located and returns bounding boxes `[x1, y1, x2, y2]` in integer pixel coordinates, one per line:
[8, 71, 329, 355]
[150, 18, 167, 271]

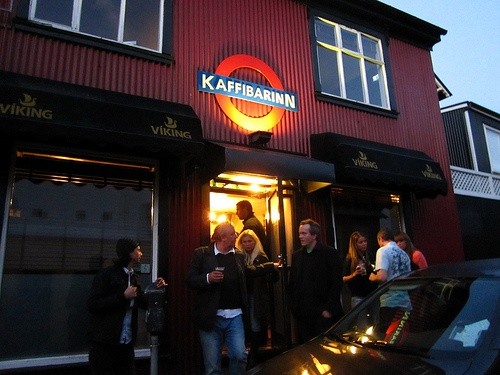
[214, 266, 225, 282]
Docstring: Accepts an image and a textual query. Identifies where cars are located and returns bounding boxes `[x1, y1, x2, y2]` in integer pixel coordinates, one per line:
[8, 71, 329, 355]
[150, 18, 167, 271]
[242, 255, 500, 375]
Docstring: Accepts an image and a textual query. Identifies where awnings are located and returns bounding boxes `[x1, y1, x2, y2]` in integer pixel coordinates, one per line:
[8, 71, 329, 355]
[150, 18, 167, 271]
[309, 131, 449, 199]
[0, 68, 204, 174]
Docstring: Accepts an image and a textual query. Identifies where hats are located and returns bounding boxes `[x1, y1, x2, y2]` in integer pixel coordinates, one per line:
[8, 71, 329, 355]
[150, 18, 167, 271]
[117, 238, 139, 255]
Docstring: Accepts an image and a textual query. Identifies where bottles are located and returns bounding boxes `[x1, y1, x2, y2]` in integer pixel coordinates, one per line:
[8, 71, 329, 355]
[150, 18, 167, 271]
[361, 264, 366, 275]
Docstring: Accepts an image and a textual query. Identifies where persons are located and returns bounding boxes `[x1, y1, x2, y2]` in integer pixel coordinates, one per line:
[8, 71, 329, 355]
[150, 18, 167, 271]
[86, 238, 168, 375]
[341, 231, 376, 308]
[369, 230, 411, 284]
[394, 231, 428, 271]
[186, 201, 280, 375]
[287, 218, 344, 347]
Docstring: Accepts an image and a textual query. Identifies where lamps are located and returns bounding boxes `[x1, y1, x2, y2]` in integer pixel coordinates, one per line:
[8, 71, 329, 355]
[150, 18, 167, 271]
[248, 131, 273, 144]
